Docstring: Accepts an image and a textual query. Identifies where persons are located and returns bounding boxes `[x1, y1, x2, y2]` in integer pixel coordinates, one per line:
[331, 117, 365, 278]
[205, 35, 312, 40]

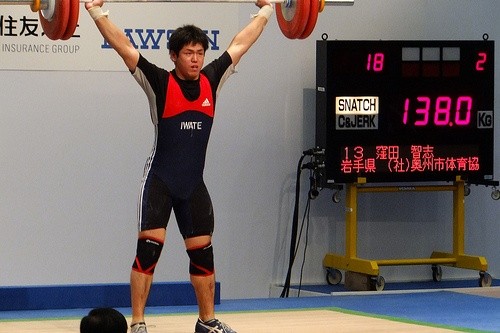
[84, 0, 274, 333]
[79, 307, 129, 333]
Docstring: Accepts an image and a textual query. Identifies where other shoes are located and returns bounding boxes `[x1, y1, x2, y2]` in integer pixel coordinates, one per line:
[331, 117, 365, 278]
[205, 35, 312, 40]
[130, 321, 147, 333]
[194, 317, 237, 333]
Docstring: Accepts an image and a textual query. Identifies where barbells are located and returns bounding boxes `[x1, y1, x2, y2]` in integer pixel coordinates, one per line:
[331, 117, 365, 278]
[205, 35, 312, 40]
[19, 0, 355, 40]
